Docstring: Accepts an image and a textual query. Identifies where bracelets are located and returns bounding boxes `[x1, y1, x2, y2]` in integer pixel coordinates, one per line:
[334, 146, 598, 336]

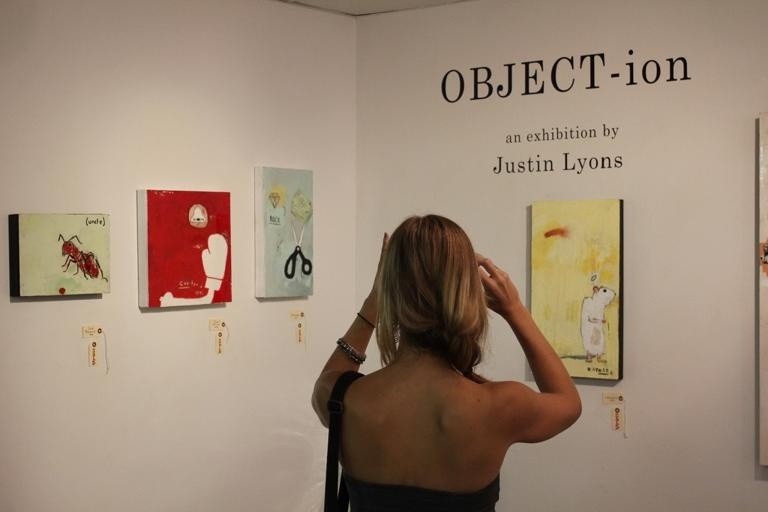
[357, 313, 376, 330]
[336, 337, 367, 365]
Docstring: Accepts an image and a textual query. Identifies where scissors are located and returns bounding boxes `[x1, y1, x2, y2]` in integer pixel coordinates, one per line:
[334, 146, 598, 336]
[285, 226, 311, 278]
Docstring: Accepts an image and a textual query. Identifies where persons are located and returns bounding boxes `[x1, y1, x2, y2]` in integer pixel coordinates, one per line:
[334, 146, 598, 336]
[310, 215, 582, 511]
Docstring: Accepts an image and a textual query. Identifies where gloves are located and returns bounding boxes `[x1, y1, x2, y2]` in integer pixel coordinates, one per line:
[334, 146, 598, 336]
[200, 232, 229, 291]
